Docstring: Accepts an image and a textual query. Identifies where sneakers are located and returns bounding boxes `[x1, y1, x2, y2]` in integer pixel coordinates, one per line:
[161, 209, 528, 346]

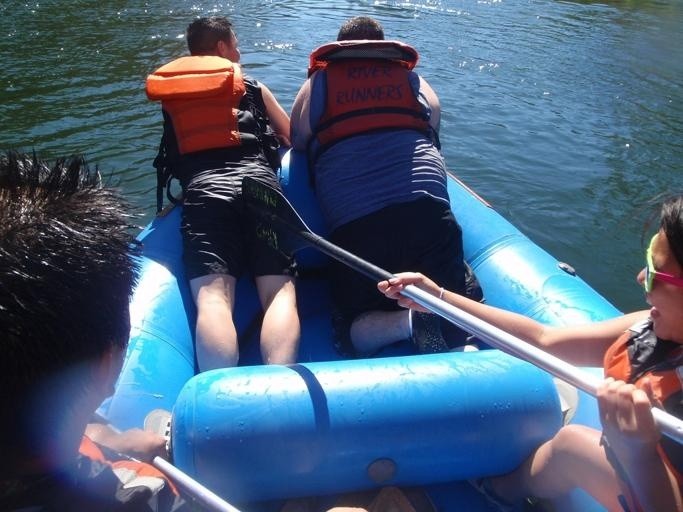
[409, 309, 447, 353]
[468, 477, 518, 511]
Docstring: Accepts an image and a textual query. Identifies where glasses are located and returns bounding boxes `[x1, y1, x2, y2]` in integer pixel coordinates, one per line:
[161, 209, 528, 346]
[645, 234, 682, 292]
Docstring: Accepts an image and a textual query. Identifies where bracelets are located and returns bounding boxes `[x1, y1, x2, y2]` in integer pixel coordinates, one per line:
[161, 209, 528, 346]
[436, 284, 444, 301]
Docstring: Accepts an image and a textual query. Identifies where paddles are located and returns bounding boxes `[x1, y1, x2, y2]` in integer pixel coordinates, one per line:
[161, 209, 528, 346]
[83, 409, 241, 512]
[242, 175, 683, 445]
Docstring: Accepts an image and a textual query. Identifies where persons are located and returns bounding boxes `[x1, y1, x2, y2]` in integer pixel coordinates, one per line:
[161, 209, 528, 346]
[1, 140, 187, 511]
[374, 195, 680, 512]
[282, 13, 487, 363]
[141, 12, 305, 372]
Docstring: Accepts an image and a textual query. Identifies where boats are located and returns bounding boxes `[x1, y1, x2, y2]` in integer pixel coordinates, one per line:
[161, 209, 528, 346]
[85, 145, 636, 512]
